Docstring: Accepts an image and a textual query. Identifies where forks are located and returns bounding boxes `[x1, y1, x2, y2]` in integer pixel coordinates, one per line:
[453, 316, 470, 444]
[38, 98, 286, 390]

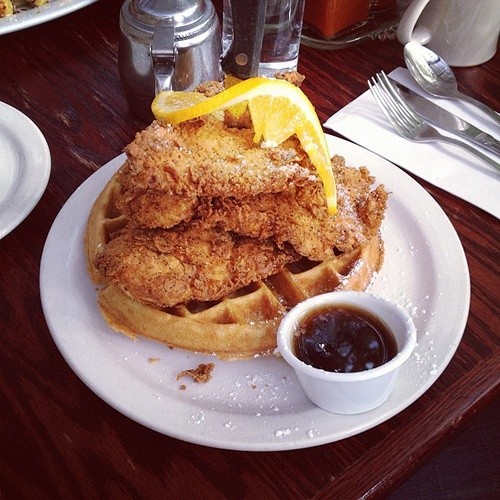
[366, 71, 500, 168]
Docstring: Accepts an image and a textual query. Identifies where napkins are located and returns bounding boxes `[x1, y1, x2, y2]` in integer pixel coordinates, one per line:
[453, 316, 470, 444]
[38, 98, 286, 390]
[322, 65, 500, 215]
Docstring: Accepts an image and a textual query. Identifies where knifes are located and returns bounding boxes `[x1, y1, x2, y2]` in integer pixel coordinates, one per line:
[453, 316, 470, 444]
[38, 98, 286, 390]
[376, 73, 500, 158]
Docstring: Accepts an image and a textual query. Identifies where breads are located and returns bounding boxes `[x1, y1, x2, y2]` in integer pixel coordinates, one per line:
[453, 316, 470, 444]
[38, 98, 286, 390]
[85, 160, 388, 362]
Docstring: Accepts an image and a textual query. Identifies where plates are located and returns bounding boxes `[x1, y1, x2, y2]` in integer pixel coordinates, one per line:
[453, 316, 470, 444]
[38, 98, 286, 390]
[0, 100, 52, 241]
[0, 0, 96, 34]
[38, 133, 471, 452]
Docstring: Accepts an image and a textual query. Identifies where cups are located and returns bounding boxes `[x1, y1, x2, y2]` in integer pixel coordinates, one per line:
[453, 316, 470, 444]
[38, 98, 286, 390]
[396, 0, 500, 67]
[220, 0, 306, 81]
[276, 291, 418, 415]
[117, 1, 223, 124]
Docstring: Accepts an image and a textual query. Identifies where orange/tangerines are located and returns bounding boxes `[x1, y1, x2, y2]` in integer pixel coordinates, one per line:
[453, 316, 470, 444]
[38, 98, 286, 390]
[150, 75, 337, 219]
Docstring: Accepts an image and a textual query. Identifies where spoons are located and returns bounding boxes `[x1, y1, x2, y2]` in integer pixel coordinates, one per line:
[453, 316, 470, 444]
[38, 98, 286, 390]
[403, 41, 500, 127]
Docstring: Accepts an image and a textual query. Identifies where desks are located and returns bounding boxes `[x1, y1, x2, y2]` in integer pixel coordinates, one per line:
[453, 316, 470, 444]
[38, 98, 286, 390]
[0, 0, 500, 500]
